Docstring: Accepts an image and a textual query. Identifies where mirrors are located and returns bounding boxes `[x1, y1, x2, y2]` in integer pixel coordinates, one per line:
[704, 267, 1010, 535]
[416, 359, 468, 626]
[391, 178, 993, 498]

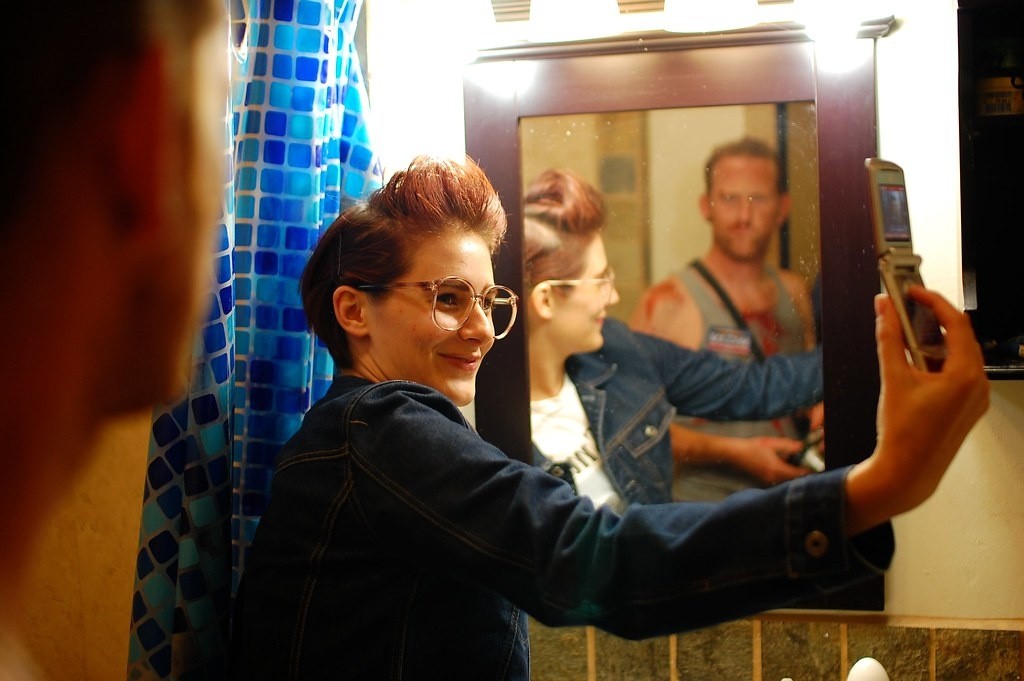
[460, 14, 898, 611]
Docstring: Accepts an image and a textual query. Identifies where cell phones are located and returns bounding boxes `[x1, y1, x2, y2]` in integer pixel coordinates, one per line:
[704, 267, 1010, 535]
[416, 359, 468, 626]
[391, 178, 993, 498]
[865, 157, 946, 373]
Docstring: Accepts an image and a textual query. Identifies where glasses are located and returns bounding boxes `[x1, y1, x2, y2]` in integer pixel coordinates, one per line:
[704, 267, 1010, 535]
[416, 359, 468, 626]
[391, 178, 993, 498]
[529, 266, 615, 308]
[356, 275, 519, 339]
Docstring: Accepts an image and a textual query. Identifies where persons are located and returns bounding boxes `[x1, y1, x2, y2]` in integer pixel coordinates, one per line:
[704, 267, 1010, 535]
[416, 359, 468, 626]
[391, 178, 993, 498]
[226, 154, 990, 681]
[0, 1, 226, 681]
[630, 136, 824, 502]
[522, 167, 823, 518]
